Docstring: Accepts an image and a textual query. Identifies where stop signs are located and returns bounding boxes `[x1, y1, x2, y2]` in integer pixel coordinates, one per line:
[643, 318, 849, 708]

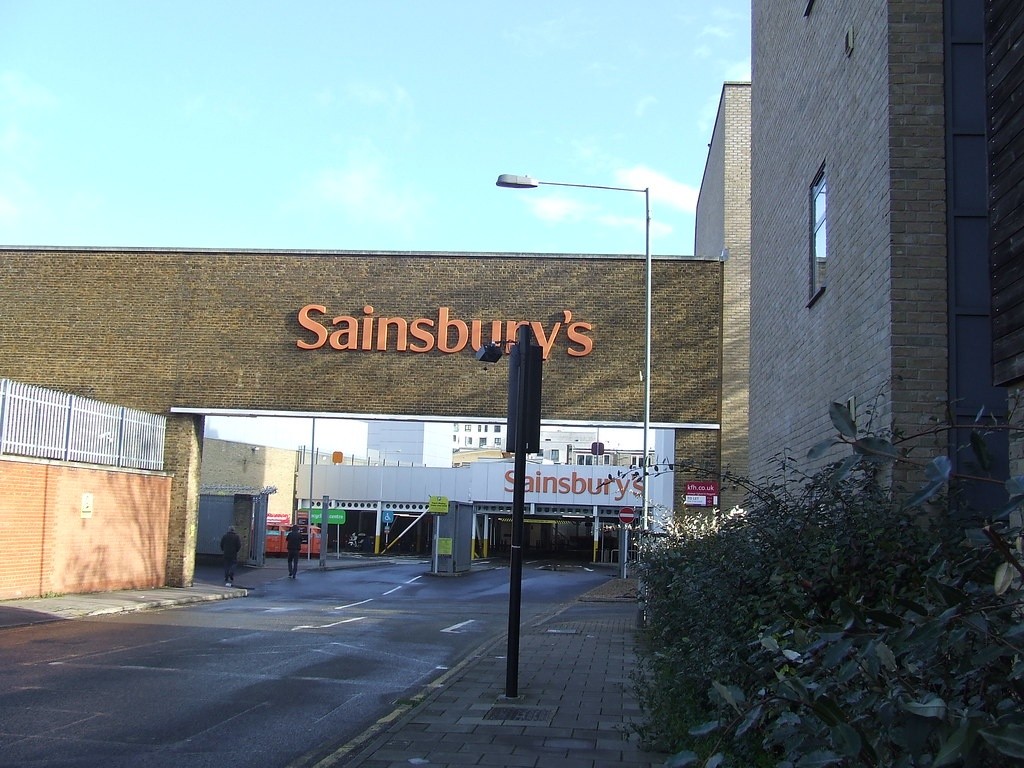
[619, 507, 635, 524]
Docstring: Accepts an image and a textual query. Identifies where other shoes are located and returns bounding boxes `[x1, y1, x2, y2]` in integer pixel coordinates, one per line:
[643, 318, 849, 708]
[224, 577, 234, 582]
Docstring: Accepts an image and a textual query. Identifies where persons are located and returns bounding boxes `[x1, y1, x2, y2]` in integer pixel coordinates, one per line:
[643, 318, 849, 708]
[285, 526, 302, 579]
[220, 525, 240, 582]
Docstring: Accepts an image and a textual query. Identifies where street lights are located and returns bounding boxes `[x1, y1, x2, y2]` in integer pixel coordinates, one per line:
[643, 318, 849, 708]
[496, 174, 652, 629]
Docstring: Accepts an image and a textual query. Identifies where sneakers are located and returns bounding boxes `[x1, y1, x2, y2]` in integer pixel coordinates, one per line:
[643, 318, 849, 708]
[288, 572, 296, 580]
